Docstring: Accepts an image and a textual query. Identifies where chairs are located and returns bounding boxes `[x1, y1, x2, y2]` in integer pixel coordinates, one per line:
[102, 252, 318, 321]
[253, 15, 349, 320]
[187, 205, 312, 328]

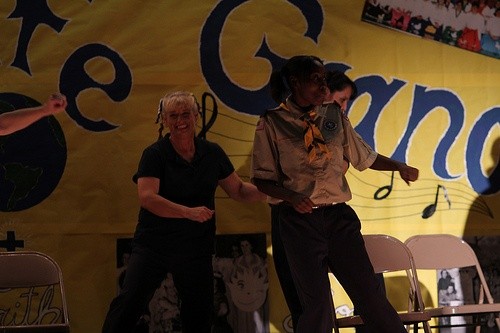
[0, 250, 71, 333]
[403, 233, 500, 333]
[329, 234, 433, 333]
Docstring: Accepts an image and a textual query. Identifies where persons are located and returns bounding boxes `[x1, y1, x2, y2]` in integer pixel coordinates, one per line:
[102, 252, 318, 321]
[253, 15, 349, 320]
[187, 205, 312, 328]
[268, 71, 357, 333]
[251, 55, 418, 333]
[365, 0, 500, 58]
[463, 139, 500, 303]
[232, 239, 263, 272]
[0, 91, 67, 135]
[102, 91, 284, 333]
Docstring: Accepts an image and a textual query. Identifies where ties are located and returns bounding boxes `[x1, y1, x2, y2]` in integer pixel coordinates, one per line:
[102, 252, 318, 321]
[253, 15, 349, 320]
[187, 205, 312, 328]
[280, 102, 331, 164]
